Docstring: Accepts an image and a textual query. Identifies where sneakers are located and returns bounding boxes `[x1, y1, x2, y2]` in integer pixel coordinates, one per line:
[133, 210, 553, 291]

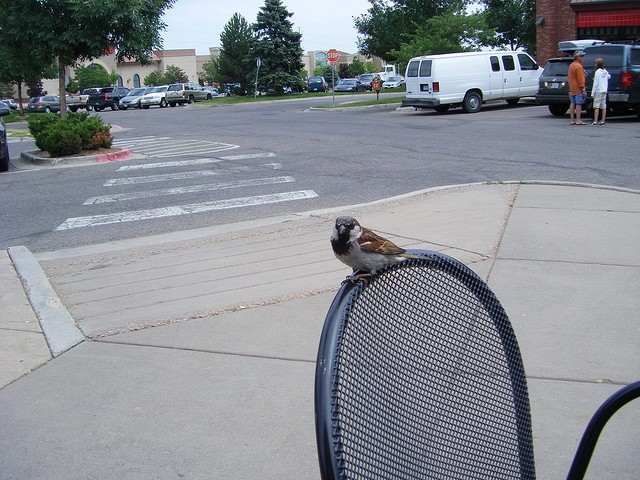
[599, 121, 605, 125]
[592, 121, 597, 126]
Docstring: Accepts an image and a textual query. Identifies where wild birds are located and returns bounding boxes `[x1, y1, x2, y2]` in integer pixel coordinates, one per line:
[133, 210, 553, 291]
[330, 214, 436, 286]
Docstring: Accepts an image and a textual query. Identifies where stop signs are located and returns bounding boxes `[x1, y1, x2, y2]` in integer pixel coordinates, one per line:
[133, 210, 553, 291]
[326, 48, 340, 62]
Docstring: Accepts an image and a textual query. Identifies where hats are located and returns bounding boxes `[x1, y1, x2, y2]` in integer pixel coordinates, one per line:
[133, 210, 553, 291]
[573, 51, 586, 60]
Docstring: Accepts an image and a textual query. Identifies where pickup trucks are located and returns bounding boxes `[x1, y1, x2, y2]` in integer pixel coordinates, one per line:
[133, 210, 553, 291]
[66, 88, 102, 111]
[91, 87, 130, 112]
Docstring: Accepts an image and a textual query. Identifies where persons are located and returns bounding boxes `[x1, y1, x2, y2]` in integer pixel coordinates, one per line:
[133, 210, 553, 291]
[591, 57, 612, 125]
[568, 51, 587, 125]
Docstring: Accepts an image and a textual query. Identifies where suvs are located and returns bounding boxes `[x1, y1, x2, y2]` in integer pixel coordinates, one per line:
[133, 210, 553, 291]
[579, 39, 640, 120]
[535, 40, 605, 116]
[165, 83, 213, 107]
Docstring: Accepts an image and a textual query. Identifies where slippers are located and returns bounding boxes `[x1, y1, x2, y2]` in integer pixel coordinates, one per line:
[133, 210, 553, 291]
[576, 121, 586, 125]
[571, 121, 576, 125]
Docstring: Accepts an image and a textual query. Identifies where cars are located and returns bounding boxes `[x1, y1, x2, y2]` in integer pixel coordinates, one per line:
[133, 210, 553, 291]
[28, 96, 60, 113]
[334, 79, 361, 92]
[382, 76, 405, 90]
[359, 74, 380, 91]
[0, 118, 10, 171]
[141, 85, 169, 109]
[307, 76, 329, 91]
[0, 98, 17, 116]
[119, 87, 147, 110]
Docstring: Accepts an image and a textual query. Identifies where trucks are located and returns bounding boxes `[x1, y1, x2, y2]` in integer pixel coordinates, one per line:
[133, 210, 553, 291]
[402, 50, 543, 113]
